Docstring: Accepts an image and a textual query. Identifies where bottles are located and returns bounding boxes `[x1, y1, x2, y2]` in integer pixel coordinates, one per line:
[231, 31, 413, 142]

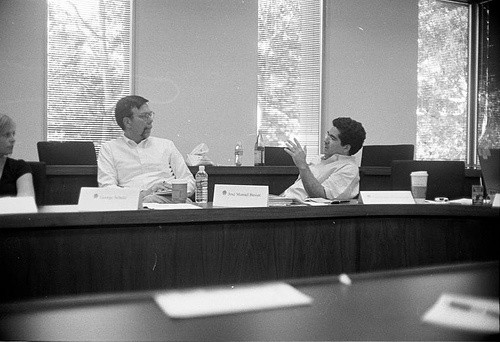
[195, 166, 208, 203]
[254, 130, 266, 166]
[235, 141, 244, 165]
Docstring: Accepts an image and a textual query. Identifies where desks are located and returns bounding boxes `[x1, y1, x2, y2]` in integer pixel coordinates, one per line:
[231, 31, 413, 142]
[0, 161, 500, 342]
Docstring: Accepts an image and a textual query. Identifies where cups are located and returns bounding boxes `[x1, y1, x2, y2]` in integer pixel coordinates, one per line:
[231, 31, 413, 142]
[472, 185, 484, 205]
[410, 170, 428, 203]
[172, 179, 187, 203]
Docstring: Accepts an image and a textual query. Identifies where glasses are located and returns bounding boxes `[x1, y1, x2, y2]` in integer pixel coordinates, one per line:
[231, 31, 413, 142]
[133, 110, 153, 120]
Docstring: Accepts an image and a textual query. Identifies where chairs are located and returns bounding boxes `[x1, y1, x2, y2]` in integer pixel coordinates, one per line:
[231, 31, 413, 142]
[37, 141, 97, 165]
[265, 146, 295, 166]
[361, 144, 414, 165]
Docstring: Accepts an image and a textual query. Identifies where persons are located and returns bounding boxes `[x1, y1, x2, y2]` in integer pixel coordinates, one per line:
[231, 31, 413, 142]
[0, 114, 46, 207]
[279, 117, 366, 202]
[97, 96, 197, 203]
